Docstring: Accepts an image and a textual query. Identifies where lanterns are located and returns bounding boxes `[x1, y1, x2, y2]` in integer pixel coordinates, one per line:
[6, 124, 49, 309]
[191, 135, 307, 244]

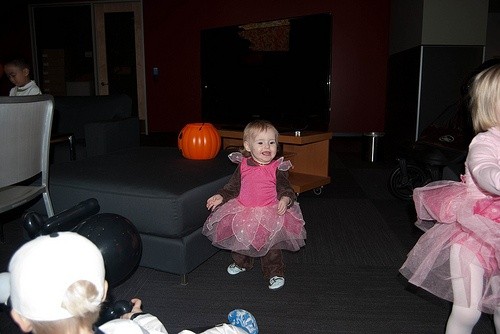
[177, 122, 220, 159]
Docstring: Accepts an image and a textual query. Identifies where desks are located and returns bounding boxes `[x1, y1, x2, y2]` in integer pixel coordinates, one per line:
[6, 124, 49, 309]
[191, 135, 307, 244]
[218, 130, 332, 194]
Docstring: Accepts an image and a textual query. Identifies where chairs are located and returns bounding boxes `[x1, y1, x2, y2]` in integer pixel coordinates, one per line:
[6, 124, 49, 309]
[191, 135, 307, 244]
[0, 96, 56, 218]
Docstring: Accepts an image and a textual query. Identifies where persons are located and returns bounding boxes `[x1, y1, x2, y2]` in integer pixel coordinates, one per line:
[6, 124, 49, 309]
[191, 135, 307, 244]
[3, 54, 43, 97]
[200, 119, 308, 290]
[8, 231, 259, 334]
[444, 63, 500, 334]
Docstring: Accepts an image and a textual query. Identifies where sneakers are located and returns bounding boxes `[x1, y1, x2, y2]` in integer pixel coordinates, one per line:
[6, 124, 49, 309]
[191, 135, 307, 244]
[269, 277, 284, 290]
[227, 262, 245, 275]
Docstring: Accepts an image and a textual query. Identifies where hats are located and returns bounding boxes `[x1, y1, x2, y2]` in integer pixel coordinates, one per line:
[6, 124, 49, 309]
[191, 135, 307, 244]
[9, 232, 105, 322]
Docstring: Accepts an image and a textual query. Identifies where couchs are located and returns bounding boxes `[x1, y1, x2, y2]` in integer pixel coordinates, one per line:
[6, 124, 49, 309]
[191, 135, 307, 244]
[25, 94, 236, 286]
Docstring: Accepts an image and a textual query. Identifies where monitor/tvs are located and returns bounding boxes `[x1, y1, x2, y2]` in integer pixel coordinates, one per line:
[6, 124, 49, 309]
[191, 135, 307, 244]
[200, 12, 333, 132]
